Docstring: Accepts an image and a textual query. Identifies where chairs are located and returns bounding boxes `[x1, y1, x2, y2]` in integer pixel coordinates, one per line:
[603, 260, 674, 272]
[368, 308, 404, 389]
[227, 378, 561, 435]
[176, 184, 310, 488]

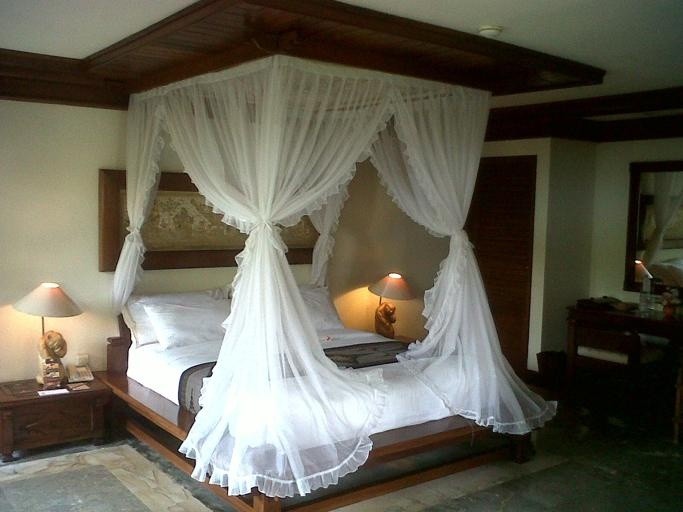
[565, 319, 671, 422]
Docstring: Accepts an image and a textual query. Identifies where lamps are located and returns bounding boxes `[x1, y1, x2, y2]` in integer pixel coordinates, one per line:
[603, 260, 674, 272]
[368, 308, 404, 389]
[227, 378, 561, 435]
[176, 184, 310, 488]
[635, 259, 655, 279]
[13, 281, 84, 385]
[366, 271, 419, 338]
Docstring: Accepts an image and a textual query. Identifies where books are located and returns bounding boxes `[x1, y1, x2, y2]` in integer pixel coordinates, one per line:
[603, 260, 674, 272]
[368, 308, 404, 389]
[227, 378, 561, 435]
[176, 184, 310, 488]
[35, 389, 69, 398]
[65, 382, 90, 392]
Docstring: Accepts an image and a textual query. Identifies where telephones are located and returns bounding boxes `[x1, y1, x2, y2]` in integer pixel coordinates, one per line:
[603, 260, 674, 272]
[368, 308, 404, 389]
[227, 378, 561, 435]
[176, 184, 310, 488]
[65, 363, 94, 384]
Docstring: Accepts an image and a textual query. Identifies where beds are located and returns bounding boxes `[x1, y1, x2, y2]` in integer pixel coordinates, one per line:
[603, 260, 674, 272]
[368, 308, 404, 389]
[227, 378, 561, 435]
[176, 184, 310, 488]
[91, 310, 539, 512]
[637, 256, 682, 284]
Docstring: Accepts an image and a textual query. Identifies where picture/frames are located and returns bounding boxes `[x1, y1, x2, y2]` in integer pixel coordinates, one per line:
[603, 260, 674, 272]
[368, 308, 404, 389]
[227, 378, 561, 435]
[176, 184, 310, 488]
[96, 166, 317, 273]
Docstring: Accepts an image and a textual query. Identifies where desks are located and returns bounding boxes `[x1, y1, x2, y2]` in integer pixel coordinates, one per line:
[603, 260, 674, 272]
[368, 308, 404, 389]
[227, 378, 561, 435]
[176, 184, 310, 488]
[565, 306, 683, 373]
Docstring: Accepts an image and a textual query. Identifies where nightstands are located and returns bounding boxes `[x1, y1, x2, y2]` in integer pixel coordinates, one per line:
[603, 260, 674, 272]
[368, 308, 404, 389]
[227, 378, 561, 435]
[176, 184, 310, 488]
[0, 375, 114, 462]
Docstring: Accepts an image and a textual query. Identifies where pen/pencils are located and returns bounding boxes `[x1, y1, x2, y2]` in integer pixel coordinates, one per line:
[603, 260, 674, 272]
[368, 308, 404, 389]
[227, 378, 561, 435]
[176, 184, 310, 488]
[70, 381, 89, 390]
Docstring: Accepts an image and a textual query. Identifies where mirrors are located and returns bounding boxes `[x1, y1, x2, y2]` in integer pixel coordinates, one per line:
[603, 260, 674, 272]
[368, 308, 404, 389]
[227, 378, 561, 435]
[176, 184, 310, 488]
[621, 158, 682, 298]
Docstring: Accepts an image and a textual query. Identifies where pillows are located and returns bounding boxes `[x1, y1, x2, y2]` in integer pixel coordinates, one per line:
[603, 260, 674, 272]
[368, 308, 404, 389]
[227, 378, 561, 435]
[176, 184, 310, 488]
[300, 285, 347, 330]
[122, 284, 231, 347]
[142, 299, 236, 351]
